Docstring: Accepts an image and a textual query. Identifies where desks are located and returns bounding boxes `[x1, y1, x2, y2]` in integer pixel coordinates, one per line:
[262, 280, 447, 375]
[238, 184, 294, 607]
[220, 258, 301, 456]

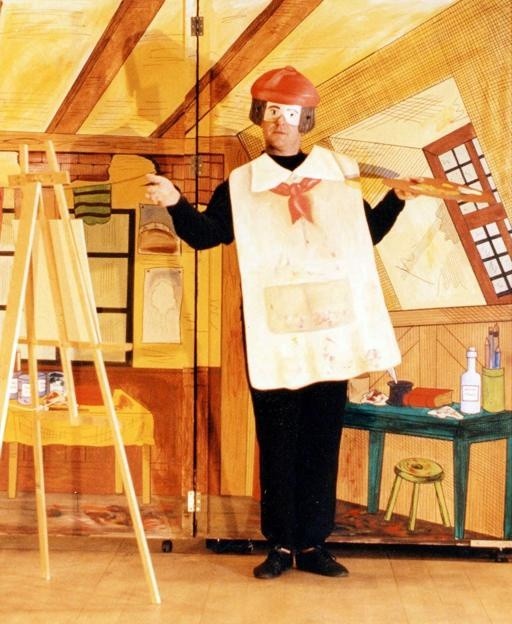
[344, 402, 512, 540]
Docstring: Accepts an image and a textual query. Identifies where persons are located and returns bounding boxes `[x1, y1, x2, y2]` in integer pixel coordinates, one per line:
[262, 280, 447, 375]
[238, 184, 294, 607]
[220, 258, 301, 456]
[141, 65, 423, 581]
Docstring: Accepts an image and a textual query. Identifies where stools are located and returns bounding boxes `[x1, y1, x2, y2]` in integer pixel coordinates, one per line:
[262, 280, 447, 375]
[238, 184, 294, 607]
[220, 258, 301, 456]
[383, 457, 453, 532]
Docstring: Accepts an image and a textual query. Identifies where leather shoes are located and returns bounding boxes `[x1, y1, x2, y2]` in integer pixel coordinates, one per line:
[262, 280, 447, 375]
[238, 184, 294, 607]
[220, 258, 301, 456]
[253, 548, 294, 579]
[296, 546, 348, 576]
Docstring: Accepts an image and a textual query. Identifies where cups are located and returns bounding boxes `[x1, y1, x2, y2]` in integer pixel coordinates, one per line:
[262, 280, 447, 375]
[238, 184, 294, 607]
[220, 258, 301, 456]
[481, 366, 507, 415]
[10, 367, 66, 407]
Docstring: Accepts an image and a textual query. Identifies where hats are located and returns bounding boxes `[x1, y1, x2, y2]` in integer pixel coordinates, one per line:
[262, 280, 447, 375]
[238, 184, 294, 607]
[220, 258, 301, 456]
[251, 67, 321, 106]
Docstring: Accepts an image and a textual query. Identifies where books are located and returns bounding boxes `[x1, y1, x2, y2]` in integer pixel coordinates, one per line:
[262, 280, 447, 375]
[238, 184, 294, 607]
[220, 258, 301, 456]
[403, 384, 456, 408]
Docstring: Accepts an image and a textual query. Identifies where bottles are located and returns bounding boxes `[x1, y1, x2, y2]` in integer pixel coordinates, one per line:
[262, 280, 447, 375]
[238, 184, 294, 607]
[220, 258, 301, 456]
[459, 346, 481, 415]
